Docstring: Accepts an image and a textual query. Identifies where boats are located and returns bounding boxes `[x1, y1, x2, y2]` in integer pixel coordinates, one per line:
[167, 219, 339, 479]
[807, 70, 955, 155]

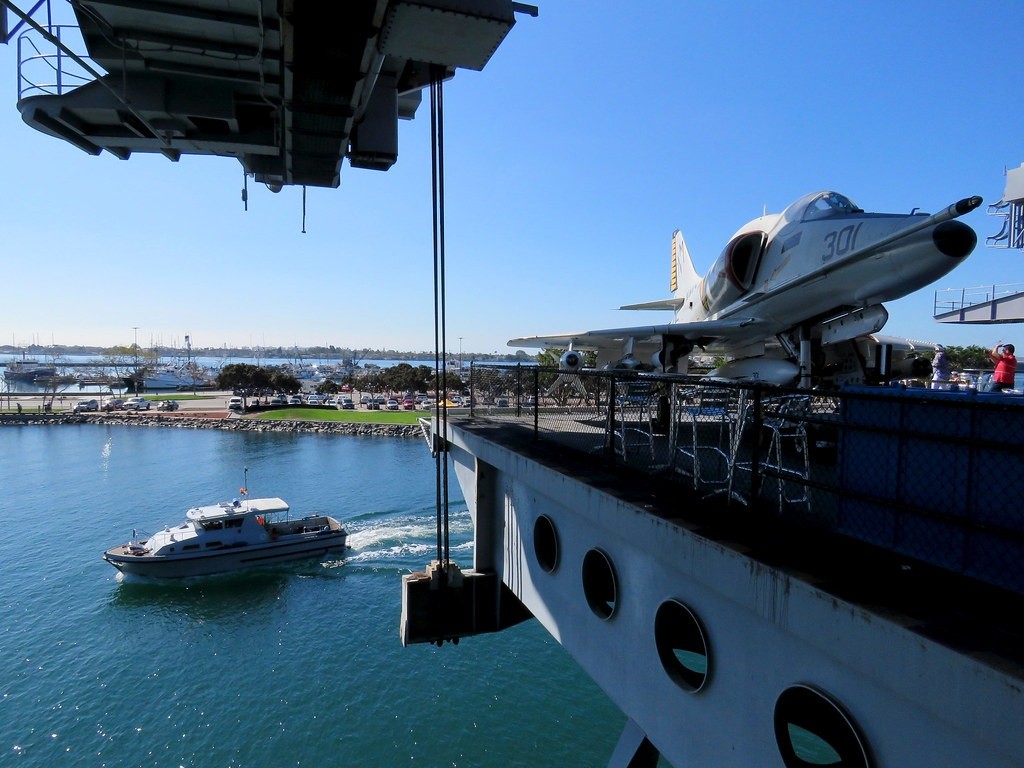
[4, 352, 57, 382]
[103, 465, 348, 583]
[32, 371, 143, 388]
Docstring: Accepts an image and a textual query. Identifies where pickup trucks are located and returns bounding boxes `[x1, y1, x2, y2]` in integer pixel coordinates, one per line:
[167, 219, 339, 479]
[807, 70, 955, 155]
[122, 396, 151, 411]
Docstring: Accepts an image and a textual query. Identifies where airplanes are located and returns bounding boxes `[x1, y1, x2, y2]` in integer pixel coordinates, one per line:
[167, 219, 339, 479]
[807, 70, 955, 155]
[505, 189, 983, 392]
[541, 350, 598, 397]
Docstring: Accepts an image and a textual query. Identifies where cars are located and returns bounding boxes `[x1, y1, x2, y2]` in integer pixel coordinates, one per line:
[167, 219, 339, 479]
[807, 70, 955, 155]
[157, 400, 179, 411]
[228, 383, 536, 410]
[74, 399, 98, 412]
[100, 399, 124, 411]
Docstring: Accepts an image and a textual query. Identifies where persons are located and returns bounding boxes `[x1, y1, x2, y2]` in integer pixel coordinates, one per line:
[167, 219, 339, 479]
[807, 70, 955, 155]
[985, 344, 1016, 393]
[929, 344, 950, 391]
[949, 371, 961, 381]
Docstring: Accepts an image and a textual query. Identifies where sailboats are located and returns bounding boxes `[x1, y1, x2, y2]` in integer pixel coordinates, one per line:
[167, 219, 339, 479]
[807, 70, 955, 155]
[134, 333, 348, 388]
